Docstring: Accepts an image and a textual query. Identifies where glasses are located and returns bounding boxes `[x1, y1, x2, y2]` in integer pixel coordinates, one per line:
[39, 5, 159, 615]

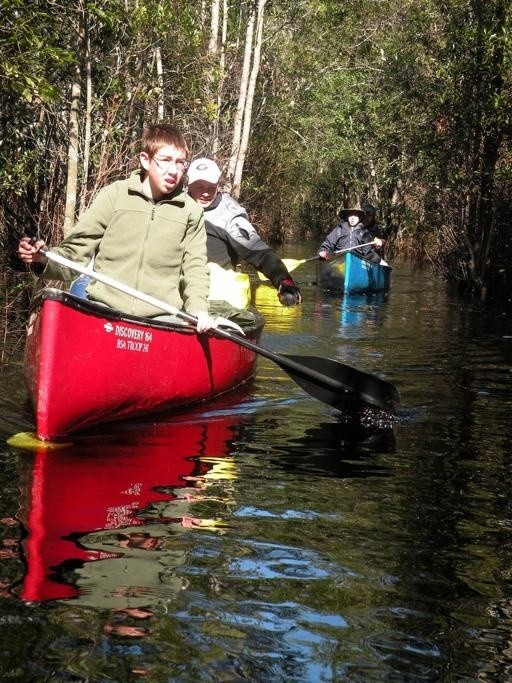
[151, 157, 190, 169]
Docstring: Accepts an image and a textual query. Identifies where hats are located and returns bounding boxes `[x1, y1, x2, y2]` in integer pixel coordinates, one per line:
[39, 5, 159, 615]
[339, 209, 367, 222]
[187, 158, 221, 185]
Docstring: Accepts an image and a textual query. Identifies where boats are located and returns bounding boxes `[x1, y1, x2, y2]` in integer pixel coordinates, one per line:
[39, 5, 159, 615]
[23, 286, 268, 445]
[315, 249, 392, 296]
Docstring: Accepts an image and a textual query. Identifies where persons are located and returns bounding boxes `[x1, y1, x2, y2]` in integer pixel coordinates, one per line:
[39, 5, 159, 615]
[14, 122, 213, 337]
[182, 150, 302, 325]
[318, 199, 387, 266]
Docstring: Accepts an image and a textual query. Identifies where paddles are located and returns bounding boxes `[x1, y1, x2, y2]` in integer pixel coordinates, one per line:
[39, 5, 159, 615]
[27, 235, 401, 422]
[256, 236, 378, 281]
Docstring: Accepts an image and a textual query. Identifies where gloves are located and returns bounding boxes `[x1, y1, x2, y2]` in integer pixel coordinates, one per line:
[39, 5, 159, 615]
[277, 279, 302, 306]
